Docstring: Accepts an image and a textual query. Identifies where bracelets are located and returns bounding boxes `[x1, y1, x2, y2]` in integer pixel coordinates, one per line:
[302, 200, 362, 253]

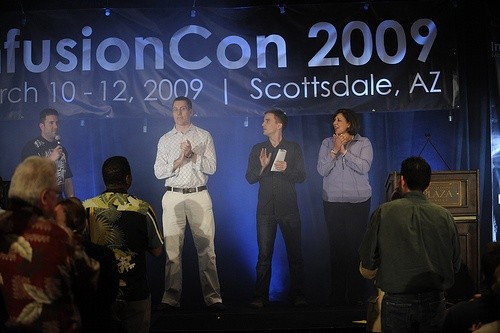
[342, 149, 348, 157]
[330, 149, 338, 156]
[186, 152, 195, 159]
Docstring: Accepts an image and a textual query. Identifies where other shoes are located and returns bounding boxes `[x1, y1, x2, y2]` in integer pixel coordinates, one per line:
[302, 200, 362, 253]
[285, 295, 308, 307]
[247, 298, 269, 309]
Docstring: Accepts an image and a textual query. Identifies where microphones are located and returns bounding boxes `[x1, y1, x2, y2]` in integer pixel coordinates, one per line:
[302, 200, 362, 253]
[55, 135, 61, 146]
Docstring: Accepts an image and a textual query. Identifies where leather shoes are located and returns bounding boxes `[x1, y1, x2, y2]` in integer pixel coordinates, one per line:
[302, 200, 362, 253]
[154, 303, 174, 312]
[209, 303, 225, 312]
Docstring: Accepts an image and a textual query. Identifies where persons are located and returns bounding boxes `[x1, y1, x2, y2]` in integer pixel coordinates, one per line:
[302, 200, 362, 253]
[154, 96, 230, 312]
[359, 155, 462, 333]
[22, 109, 73, 203]
[0, 156, 81, 333]
[317, 109, 373, 308]
[55, 197, 120, 333]
[82, 156, 163, 333]
[245, 109, 310, 308]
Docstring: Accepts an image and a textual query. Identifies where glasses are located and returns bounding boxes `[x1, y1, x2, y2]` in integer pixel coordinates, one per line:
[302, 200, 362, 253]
[172, 106, 189, 111]
[48, 188, 62, 197]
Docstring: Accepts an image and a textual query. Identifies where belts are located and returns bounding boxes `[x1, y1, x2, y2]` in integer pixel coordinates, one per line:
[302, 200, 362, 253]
[166, 185, 207, 194]
